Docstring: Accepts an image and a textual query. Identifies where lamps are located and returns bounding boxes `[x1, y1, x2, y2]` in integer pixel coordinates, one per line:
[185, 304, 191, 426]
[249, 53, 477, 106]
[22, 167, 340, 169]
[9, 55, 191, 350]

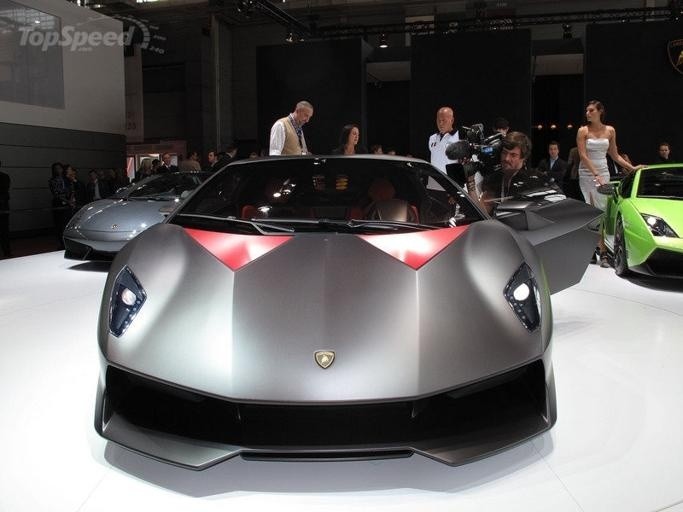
[378, 34, 391, 50]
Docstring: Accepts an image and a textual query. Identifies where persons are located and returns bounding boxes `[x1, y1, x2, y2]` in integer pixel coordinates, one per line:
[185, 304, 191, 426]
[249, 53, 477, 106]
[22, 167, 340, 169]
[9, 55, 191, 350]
[0, 171, 11, 239]
[331, 124, 365, 156]
[606, 145, 629, 177]
[373, 106, 534, 217]
[568, 147, 580, 199]
[268, 101, 314, 157]
[47, 142, 237, 242]
[537, 141, 567, 192]
[655, 143, 675, 164]
[575, 100, 648, 212]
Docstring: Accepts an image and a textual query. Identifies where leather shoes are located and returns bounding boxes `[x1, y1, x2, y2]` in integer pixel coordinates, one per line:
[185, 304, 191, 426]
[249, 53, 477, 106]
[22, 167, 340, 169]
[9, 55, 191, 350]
[600, 252, 610, 268]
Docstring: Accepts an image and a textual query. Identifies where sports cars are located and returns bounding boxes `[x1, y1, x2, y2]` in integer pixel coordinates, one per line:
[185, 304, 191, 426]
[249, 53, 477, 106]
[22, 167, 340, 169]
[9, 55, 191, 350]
[94, 152, 607, 473]
[597, 163, 682, 281]
[60, 168, 212, 265]
